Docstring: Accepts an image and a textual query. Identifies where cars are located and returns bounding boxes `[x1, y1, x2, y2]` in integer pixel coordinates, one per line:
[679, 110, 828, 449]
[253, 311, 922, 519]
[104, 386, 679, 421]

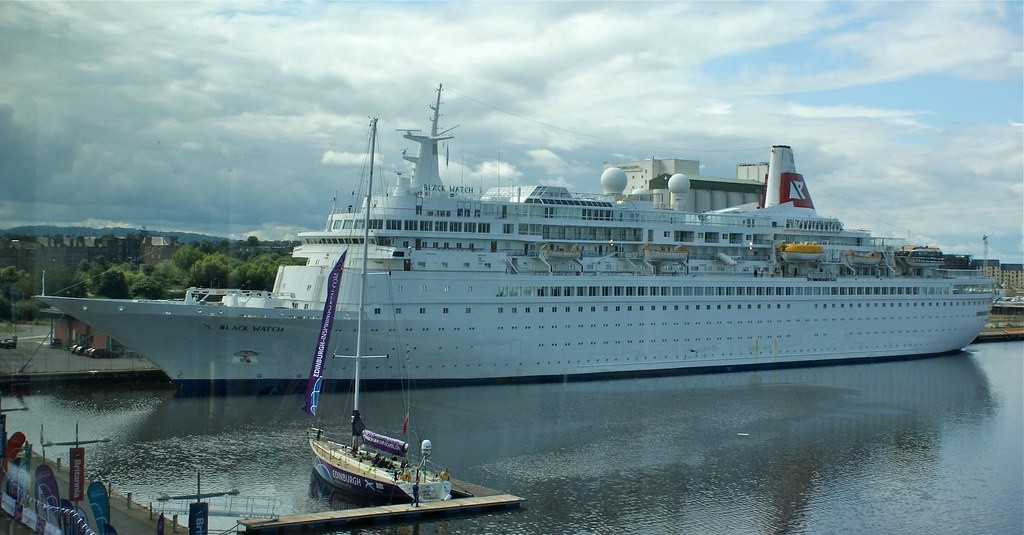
[0, 339, 17, 348]
[83, 348, 96, 355]
[70, 344, 82, 353]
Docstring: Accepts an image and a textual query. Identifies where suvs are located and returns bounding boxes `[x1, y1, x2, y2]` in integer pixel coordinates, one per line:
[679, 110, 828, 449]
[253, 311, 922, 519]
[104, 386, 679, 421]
[75, 345, 91, 354]
[90, 348, 118, 359]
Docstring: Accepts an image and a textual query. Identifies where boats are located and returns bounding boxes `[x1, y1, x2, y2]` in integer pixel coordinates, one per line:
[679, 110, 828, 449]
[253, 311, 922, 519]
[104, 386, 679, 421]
[539, 242, 582, 260]
[846, 250, 881, 265]
[780, 241, 824, 262]
[642, 244, 689, 261]
[896, 245, 946, 267]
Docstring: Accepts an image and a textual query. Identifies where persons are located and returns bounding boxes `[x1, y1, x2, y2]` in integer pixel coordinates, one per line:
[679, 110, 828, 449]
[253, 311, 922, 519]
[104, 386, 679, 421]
[394, 459, 410, 480]
[440, 471, 448, 480]
[371, 454, 396, 469]
[754, 270, 764, 277]
[411, 482, 420, 507]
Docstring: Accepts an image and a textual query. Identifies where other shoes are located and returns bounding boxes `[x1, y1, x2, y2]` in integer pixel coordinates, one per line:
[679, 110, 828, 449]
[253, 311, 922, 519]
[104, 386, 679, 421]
[411, 503, 413, 506]
[416, 506, 420, 507]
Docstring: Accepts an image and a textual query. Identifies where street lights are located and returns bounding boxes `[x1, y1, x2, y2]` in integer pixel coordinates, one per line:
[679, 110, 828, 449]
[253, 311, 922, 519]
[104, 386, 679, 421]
[156, 467, 241, 535]
[42, 420, 112, 512]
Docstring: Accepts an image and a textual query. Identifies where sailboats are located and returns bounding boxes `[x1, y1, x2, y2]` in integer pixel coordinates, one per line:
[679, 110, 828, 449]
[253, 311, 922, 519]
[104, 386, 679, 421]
[301, 114, 452, 504]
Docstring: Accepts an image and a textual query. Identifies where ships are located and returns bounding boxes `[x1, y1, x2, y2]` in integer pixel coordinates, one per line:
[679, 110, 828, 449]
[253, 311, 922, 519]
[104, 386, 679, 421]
[32, 84, 998, 399]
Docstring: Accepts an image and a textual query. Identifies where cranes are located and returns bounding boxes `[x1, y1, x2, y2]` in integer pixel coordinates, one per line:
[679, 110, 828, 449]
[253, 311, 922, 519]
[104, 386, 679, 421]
[983, 232, 996, 276]
